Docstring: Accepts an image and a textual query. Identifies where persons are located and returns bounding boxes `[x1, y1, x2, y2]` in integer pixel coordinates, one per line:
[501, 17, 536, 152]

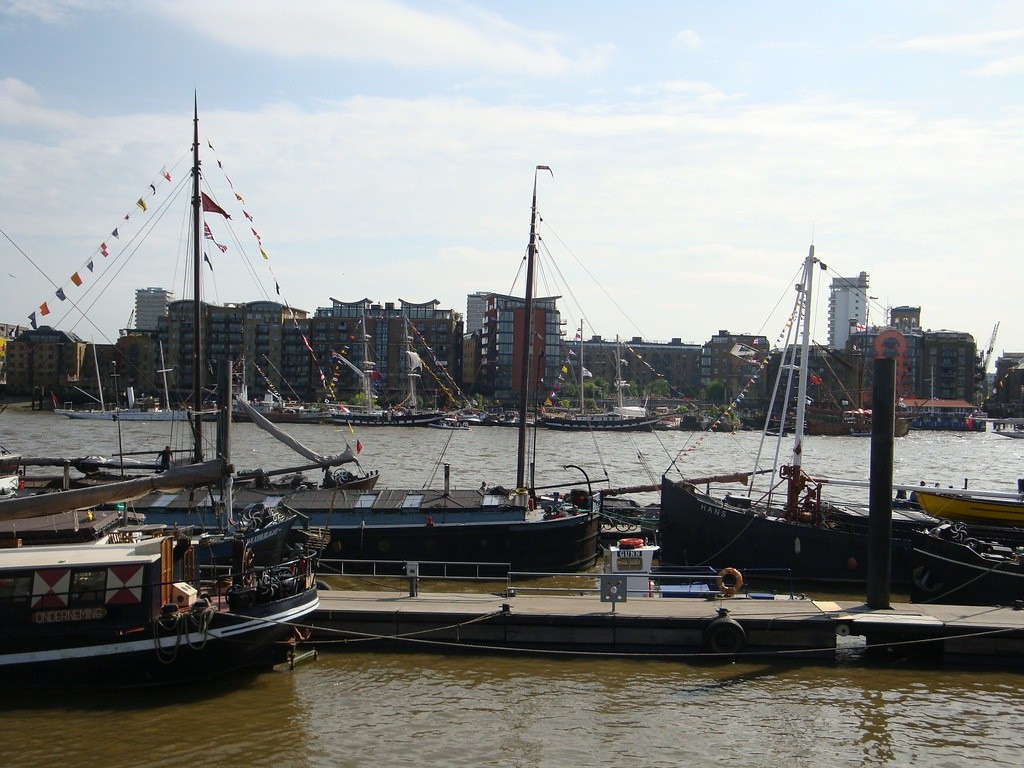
[155, 445, 173, 471]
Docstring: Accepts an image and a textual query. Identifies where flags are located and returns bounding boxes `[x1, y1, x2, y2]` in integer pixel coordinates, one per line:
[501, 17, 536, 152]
[0, 140, 282, 340]
[856, 323, 866, 332]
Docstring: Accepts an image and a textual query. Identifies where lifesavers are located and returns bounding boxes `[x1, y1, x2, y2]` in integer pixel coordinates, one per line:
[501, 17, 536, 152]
[707, 618, 746, 653]
[717, 567, 743, 593]
[324, 398, 330, 403]
[620, 538, 643, 548]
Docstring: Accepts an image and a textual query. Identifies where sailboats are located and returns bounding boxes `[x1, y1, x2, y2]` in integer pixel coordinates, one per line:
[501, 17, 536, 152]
[1, 85, 1023, 698]
